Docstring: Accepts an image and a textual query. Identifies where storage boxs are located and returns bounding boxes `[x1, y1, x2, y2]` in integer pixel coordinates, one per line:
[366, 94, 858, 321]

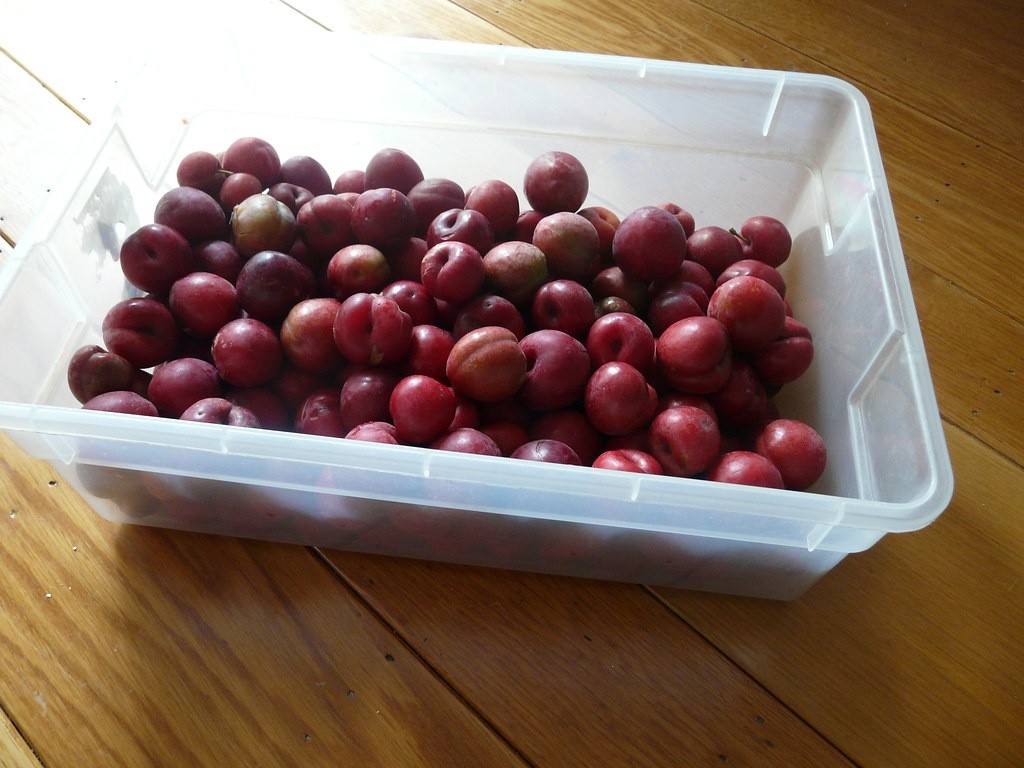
[0, 20, 954, 604]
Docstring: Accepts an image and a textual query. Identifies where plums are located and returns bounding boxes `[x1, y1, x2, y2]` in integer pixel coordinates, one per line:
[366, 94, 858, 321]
[67, 137, 826, 493]
